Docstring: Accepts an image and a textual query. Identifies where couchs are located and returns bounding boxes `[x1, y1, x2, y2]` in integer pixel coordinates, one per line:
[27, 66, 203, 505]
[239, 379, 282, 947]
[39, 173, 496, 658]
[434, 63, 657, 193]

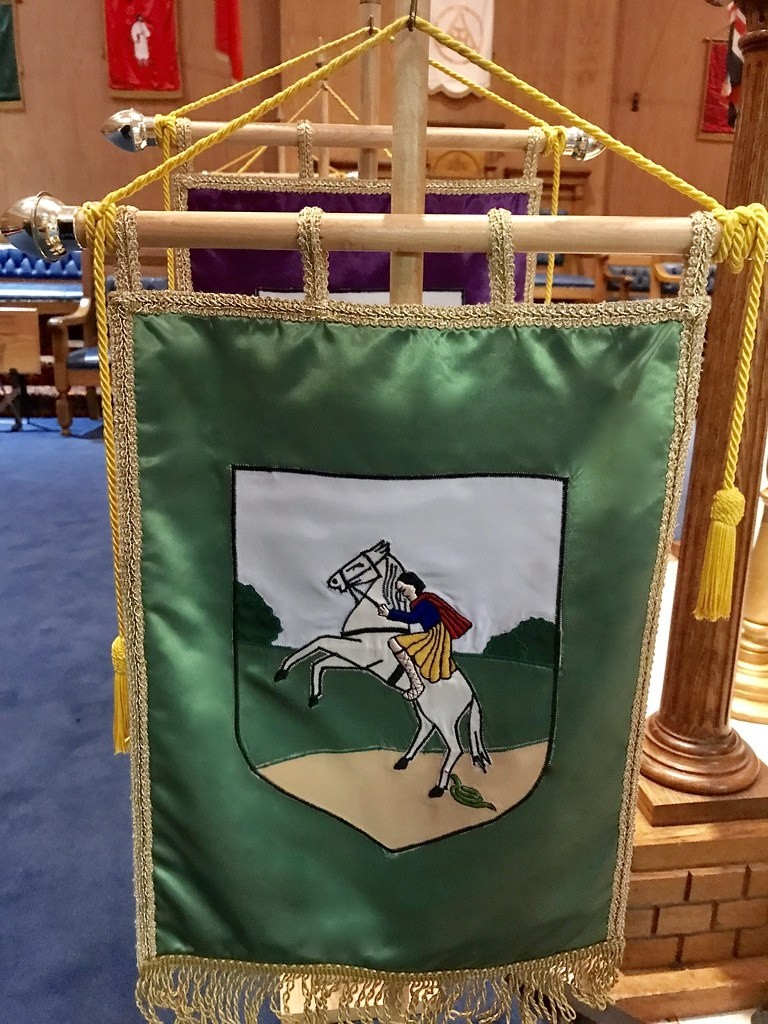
[0, 241, 86, 316]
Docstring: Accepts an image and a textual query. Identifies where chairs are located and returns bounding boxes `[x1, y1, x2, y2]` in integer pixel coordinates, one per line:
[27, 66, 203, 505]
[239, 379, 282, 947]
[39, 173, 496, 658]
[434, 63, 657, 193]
[47, 247, 170, 435]
[530, 205, 722, 304]
